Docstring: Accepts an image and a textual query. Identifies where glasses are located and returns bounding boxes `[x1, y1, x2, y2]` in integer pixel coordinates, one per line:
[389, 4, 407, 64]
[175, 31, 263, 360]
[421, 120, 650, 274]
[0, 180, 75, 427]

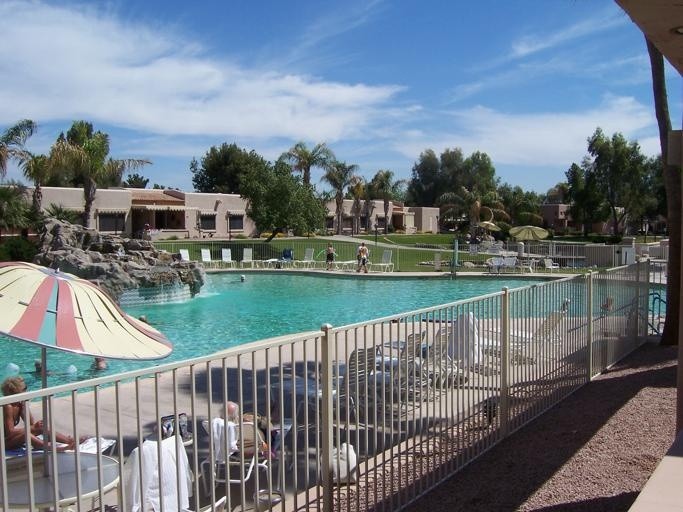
[23, 385, 28, 391]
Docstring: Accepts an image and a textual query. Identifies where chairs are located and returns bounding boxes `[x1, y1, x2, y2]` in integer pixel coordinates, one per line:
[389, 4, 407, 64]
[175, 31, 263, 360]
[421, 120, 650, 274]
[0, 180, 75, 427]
[176, 247, 395, 273]
[484, 257, 560, 273]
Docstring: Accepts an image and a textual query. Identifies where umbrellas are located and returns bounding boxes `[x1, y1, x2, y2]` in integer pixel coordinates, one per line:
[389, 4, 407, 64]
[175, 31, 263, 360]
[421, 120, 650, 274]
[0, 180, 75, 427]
[476, 220, 501, 245]
[0, 259, 174, 479]
[508, 224, 548, 265]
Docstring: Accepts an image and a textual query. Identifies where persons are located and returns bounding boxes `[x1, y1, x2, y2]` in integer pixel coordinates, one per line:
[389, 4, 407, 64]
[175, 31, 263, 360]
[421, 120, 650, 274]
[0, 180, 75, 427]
[598, 296, 614, 312]
[0, 377, 76, 452]
[208, 401, 277, 463]
[324, 242, 334, 270]
[21, 360, 51, 377]
[89, 356, 107, 370]
[355, 242, 369, 273]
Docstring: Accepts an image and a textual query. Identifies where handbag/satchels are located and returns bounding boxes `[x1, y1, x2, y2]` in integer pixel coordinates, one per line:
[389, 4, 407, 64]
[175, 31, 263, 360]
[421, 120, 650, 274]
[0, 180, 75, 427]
[160, 412, 190, 442]
[319, 439, 359, 485]
